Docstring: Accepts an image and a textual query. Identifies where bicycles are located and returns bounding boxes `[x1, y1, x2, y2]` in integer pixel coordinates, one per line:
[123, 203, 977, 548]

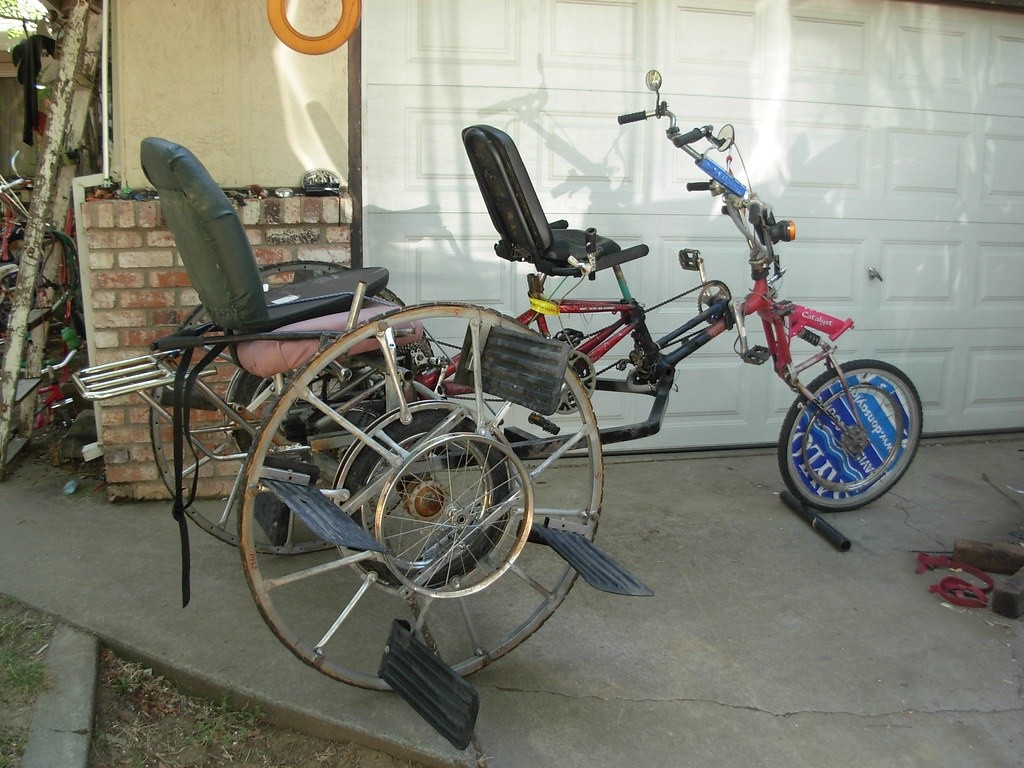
[0, 151, 78, 428]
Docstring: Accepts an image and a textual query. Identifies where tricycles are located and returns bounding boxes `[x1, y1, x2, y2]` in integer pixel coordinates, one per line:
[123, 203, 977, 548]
[68, 67, 925, 767]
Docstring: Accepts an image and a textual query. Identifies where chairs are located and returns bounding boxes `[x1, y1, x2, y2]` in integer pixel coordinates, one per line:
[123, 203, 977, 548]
[139, 133, 393, 333]
[461, 122, 622, 273]
